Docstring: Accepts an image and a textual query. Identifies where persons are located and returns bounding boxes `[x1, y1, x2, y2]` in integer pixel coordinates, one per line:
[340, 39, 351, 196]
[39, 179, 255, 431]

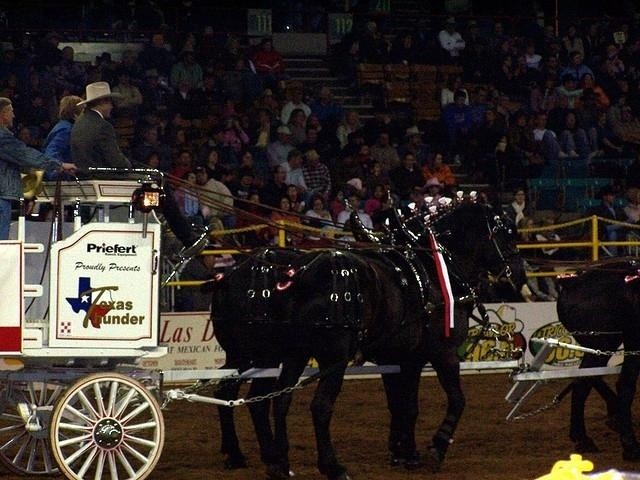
[1, 0, 639, 312]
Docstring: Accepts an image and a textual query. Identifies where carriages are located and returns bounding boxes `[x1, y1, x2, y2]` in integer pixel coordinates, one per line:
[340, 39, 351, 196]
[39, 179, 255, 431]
[0, 164, 527, 480]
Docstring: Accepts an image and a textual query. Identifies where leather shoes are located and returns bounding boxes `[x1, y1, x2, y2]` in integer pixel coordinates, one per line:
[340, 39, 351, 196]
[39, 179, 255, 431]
[181, 223, 217, 249]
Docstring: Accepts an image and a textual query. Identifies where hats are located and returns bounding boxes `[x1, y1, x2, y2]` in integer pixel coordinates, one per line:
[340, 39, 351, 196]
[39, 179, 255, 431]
[21, 170, 45, 200]
[276, 125, 293, 135]
[76, 81, 121, 107]
[425, 177, 439, 186]
[405, 125, 424, 137]
[304, 149, 321, 161]
[598, 184, 620, 194]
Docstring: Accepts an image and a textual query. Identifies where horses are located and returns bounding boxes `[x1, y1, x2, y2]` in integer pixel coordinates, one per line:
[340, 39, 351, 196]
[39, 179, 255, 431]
[200, 193, 531, 480]
[552, 258, 639, 466]
